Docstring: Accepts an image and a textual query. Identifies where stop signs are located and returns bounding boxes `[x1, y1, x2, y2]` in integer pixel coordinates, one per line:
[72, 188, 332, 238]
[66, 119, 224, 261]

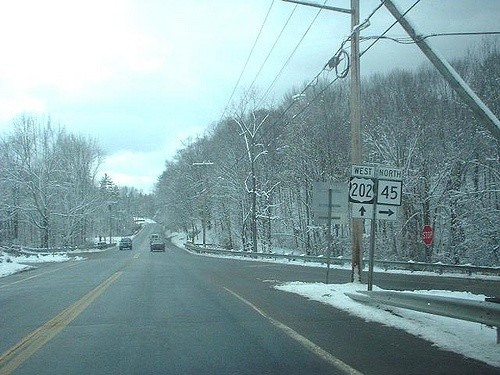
[421, 225, 434, 248]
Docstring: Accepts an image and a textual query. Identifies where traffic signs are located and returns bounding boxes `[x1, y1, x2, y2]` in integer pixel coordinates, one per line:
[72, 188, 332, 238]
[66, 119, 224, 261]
[349, 165, 378, 220]
[377, 168, 403, 220]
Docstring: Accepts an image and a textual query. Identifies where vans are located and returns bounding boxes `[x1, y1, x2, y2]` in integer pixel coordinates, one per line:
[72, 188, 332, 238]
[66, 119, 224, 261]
[150, 234, 161, 241]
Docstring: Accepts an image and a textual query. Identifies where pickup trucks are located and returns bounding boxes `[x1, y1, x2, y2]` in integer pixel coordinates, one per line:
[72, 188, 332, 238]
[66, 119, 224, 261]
[119, 238, 133, 251]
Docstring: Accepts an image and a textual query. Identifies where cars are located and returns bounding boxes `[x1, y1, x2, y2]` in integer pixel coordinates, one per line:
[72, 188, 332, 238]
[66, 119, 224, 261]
[150, 239, 166, 252]
